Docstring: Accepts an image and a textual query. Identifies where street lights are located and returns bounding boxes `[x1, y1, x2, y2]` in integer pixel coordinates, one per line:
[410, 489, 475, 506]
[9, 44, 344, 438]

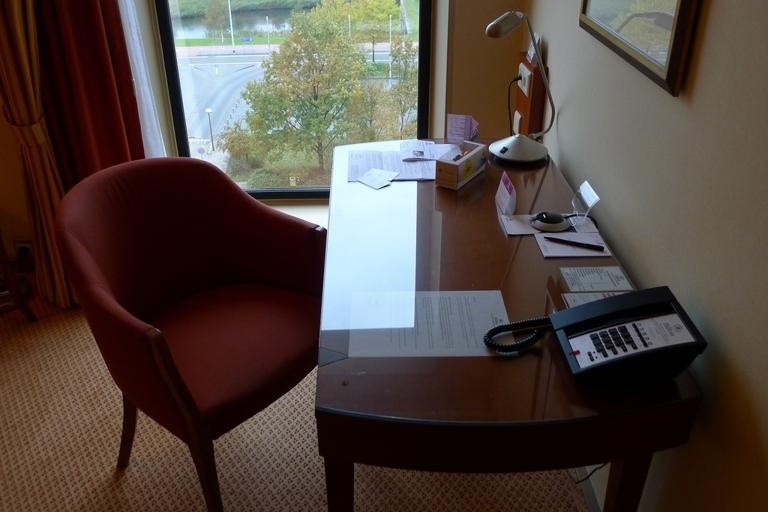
[205, 108, 214, 151]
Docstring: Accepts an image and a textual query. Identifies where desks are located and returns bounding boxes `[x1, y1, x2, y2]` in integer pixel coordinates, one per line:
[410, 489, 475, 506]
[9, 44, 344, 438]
[314, 137, 702, 512]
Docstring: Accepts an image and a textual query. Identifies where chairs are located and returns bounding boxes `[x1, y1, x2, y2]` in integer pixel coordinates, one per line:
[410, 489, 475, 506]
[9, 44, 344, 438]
[55, 156, 328, 512]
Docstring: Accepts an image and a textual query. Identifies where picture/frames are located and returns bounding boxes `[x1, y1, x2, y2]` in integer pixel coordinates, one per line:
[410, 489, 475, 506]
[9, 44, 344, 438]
[579, 0, 702, 98]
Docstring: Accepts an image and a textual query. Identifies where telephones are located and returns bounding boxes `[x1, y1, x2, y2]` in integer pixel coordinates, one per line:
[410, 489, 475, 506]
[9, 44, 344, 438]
[548, 285, 707, 384]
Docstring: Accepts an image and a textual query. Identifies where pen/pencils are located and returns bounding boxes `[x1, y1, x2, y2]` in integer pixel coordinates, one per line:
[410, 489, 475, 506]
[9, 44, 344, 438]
[544, 237, 605, 251]
[402, 158, 435, 162]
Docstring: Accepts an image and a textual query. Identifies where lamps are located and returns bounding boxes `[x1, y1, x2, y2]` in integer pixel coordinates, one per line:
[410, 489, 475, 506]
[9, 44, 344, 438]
[484, 11, 555, 171]
